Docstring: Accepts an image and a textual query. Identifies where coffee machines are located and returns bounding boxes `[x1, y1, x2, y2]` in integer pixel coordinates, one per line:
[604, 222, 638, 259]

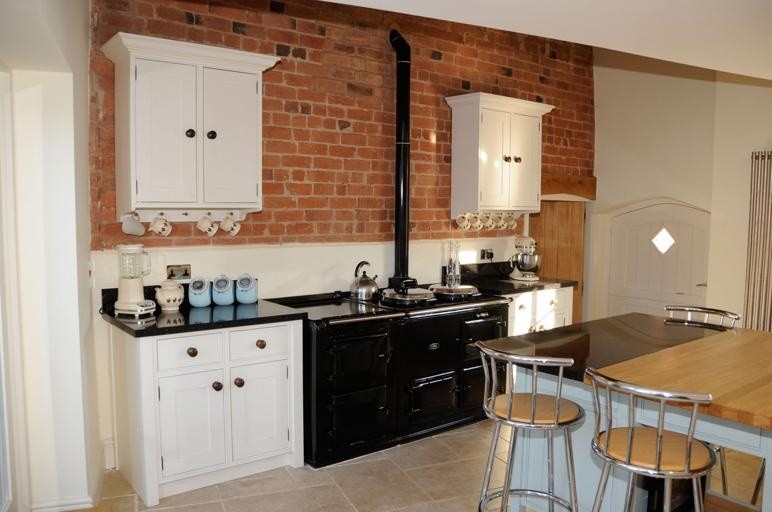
[508, 235, 542, 282]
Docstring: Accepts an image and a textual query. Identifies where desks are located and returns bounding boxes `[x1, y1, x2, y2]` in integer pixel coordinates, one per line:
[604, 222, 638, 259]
[483, 312, 772, 511]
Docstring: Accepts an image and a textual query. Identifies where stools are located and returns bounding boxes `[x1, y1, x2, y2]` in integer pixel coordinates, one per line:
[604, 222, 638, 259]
[473, 342, 586, 511]
[585, 363, 718, 510]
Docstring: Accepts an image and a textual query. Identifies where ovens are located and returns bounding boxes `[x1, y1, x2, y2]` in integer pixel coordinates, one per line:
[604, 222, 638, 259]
[389, 314, 464, 444]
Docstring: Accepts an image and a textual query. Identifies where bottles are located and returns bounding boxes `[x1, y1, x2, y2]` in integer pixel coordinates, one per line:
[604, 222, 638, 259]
[188, 273, 258, 306]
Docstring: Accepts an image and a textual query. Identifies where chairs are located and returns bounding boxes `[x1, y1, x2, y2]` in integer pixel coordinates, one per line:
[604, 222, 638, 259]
[664, 304, 740, 328]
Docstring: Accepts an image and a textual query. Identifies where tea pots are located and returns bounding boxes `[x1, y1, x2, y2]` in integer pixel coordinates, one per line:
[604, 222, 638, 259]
[349, 261, 381, 302]
[156, 277, 185, 313]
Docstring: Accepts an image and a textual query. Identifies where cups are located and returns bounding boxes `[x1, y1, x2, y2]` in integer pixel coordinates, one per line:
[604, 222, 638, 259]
[147, 216, 173, 239]
[191, 215, 219, 237]
[458, 213, 520, 232]
[217, 216, 244, 235]
[120, 217, 147, 237]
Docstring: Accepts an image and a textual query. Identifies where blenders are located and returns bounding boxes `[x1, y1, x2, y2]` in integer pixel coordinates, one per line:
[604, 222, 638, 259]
[110, 243, 157, 322]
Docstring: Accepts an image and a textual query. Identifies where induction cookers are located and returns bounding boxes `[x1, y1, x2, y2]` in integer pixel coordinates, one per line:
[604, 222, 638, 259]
[345, 295, 505, 312]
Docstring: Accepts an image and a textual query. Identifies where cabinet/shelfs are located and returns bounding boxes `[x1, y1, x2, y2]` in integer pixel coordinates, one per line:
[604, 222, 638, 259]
[506, 287, 573, 336]
[114, 55, 260, 223]
[450, 105, 541, 220]
[111, 324, 291, 506]
